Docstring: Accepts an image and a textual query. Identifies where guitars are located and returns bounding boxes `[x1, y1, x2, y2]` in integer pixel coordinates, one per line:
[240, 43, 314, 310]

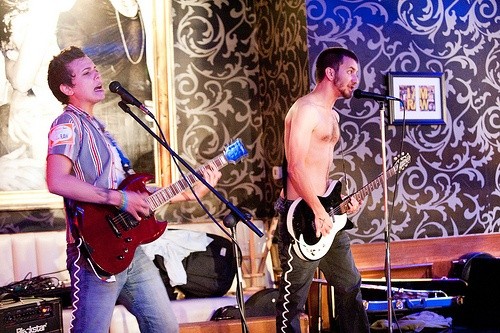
[280, 151, 412, 262]
[72, 138, 250, 285]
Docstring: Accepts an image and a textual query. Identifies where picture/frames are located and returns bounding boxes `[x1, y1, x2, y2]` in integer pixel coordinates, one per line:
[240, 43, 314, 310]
[387, 71, 446, 124]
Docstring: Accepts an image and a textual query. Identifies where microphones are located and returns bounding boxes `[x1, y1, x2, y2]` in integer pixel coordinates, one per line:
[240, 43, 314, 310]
[109, 81, 154, 118]
[353, 89, 397, 101]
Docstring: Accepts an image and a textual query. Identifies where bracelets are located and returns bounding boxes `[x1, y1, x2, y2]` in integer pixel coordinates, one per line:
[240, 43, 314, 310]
[116, 188, 129, 212]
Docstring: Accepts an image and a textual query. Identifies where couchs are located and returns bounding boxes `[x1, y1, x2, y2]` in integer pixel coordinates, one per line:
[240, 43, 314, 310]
[0, 220, 276, 333]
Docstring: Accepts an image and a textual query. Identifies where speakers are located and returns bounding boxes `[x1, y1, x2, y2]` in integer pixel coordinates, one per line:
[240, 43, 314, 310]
[0, 293, 63, 333]
[463, 258, 500, 329]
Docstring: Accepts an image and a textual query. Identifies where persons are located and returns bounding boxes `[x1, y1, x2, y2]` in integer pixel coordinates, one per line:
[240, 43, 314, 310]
[276, 46, 371, 333]
[46, 45, 179, 333]
